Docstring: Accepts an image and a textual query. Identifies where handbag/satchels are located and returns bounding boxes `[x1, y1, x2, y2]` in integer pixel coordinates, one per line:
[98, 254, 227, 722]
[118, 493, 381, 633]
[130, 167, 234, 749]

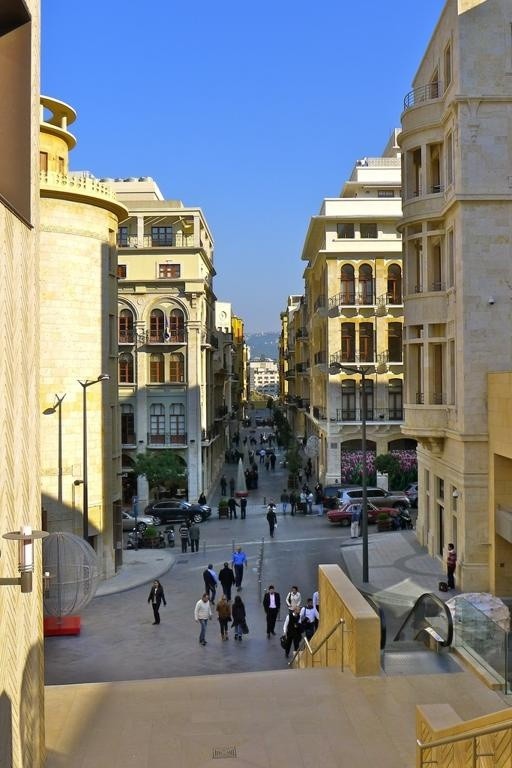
[286, 591, 291, 607]
[280, 636, 288, 649]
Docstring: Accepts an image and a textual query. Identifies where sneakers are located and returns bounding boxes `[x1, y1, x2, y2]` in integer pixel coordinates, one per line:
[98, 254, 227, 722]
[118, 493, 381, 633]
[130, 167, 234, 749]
[199, 631, 243, 646]
[271, 631, 276, 636]
[266, 633, 271, 640]
[152, 621, 160, 625]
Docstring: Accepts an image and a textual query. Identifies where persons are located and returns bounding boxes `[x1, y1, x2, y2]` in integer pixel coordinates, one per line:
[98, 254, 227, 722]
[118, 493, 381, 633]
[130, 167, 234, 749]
[148, 579, 166, 625]
[351, 506, 362, 538]
[220, 418, 325, 520]
[447, 543, 457, 589]
[390, 507, 413, 531]
[267, 508, 278, 538]
[194, 547, 319, 657]
[159, 517, 200, 552]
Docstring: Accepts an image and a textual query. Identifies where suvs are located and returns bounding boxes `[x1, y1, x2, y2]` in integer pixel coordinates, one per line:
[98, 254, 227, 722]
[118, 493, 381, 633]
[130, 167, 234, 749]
[320, 482, 418, 509]
[143, 500, 212, 526]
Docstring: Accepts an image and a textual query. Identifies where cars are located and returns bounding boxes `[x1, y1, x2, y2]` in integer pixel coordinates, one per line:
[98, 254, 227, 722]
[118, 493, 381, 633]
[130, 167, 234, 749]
[122, 508, 155, 532]
[255, 415, 264, 427]
[326, 502, 403, 527]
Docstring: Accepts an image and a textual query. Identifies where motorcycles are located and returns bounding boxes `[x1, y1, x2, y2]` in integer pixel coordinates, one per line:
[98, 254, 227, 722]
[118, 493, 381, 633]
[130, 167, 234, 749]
[127, 528, 175, 549]
[399, 507, 413, 530]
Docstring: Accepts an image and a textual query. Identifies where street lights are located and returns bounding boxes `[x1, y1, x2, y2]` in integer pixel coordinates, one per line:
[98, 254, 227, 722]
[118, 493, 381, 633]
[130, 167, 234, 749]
[72, 373, 111, 546]
[327, 362, 371, 587]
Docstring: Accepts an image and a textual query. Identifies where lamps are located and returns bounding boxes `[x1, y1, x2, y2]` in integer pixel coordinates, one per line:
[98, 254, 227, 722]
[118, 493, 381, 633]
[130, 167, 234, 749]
[0, 523, 52, 595]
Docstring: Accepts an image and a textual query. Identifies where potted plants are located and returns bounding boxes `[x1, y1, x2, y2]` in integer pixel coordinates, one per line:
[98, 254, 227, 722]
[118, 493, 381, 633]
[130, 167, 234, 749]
[138, 524, 162, 549]
[217, 497, 230, 520]
[375, 511, 393, 533]
[287, 472, 298, 490]
